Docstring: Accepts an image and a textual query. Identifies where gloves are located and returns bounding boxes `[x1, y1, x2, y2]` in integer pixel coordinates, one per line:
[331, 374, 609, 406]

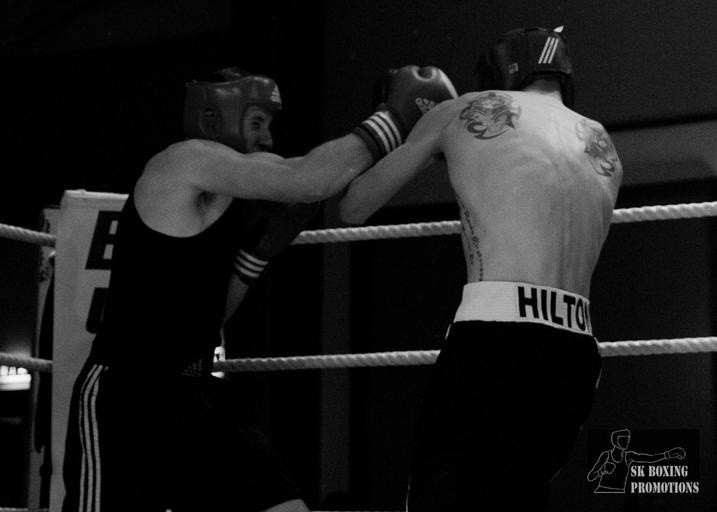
[361, 65, 457, 153]
[234, 201, 315, 272]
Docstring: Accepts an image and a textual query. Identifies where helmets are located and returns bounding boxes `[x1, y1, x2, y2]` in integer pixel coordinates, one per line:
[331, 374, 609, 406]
[185, 76, 282, 152]
[477, 25, 575, 103]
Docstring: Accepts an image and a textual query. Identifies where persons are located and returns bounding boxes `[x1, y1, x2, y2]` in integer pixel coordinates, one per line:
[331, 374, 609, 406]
[60, 66, 459, 512]
[338, 28, 623, 512]
